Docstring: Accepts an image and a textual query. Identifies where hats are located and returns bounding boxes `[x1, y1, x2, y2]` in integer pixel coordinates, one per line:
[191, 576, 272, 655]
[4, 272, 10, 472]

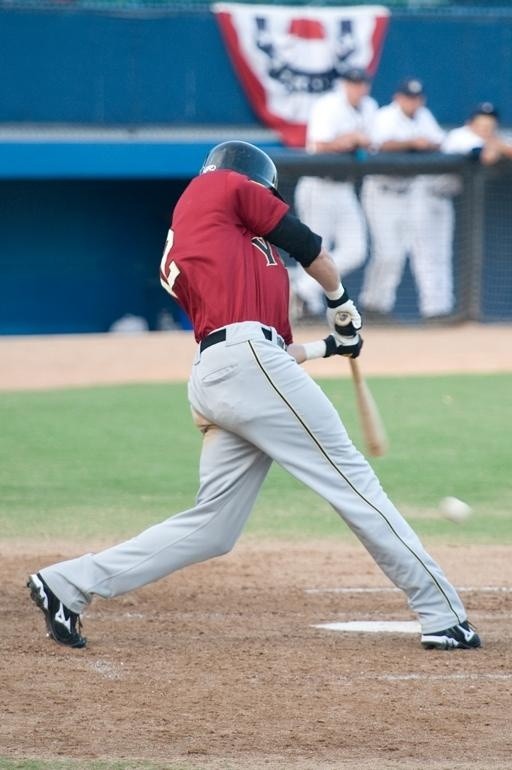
[470, 102, 498, 118]
[397, 78, 425, 95]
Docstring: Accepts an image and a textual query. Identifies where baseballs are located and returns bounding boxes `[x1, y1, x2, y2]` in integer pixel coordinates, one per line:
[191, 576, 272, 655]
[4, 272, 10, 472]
[444, 498, 470, 521]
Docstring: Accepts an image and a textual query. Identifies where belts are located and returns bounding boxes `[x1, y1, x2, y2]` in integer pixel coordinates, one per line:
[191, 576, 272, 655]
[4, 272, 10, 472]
[200, 327, 287, 353]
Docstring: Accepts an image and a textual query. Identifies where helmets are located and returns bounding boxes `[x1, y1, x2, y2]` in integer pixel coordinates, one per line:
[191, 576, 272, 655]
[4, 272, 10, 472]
[199, 140, 286, 204]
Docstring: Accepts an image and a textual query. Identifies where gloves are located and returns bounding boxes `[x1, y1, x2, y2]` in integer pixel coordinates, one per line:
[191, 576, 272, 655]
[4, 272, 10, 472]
[326, 287, 363, 361]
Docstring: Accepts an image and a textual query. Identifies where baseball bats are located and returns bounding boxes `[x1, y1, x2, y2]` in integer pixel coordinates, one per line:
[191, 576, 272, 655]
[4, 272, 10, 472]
[349, 355, 386, 455]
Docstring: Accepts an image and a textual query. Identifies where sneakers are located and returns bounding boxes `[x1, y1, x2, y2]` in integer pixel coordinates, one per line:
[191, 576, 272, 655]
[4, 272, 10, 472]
[26, 573, 88, 648]
[420, 617, 483, 651]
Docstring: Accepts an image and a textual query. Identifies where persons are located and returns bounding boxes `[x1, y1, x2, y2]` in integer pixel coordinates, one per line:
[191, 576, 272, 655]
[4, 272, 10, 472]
[26, 138, 482, 654]
[356, 79, 442, 323]
[408, 101, 512, 320]
[287, 65, 382, 319]
